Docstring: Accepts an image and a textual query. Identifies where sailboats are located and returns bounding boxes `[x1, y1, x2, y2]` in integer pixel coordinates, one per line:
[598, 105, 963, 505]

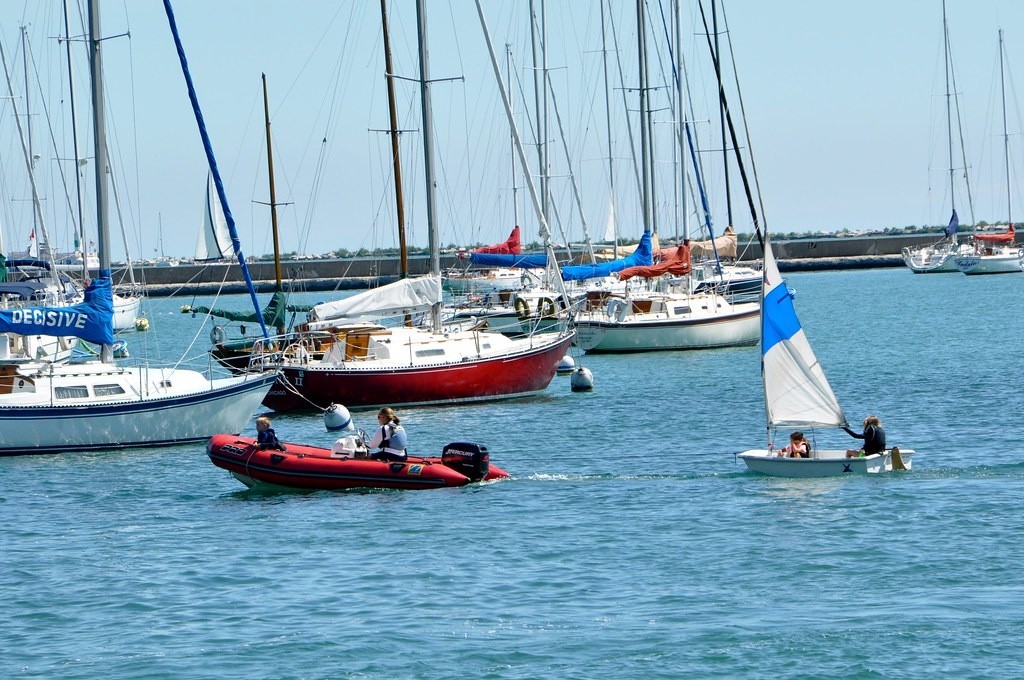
[955, 28, 1024, 275]
[732, 230, 915, 475]
[0, 0, 771, 448]
[898, 0, 978, 272]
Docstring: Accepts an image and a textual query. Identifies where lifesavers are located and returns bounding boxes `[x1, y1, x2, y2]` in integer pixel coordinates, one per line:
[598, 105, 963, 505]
[538, 297, 554, 316]
[606, 299, 627, 323]
[514, 297, 530, 317]
[210, 326, 224, 345]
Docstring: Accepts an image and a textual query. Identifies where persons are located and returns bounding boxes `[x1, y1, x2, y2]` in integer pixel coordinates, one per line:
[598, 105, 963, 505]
[255, 417, 287, 452]
[371, 407, 408, 460]
[843, 415, 886, 459]
[779, 431, 811, 458]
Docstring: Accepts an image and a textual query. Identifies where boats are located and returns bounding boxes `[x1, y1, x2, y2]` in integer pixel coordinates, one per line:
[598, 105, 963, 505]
[207, 433, 512, 492]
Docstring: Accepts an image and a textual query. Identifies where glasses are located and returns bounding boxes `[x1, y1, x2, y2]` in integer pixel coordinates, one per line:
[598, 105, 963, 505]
[377, 416, 385, 420]
